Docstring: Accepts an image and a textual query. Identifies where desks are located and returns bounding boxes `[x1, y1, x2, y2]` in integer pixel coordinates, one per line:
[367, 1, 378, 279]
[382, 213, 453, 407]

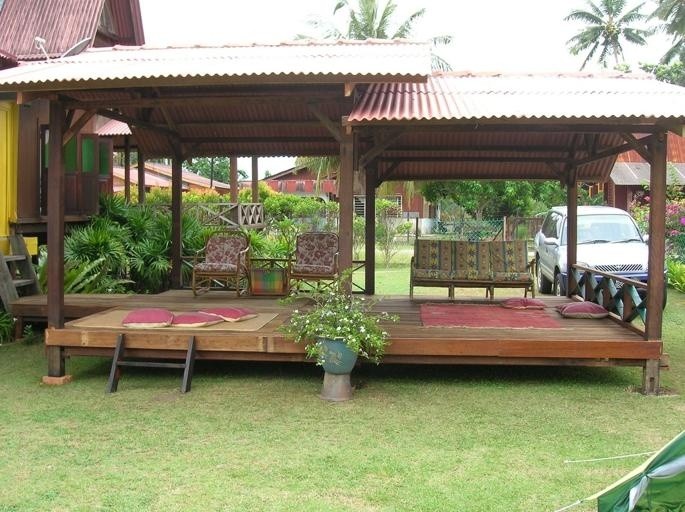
[249, 267, 287, 296]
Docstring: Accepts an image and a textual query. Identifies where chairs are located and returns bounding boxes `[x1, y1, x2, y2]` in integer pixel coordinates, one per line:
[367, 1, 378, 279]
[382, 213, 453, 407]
[192, 231, 253, 298]
[286, 231, 340, 298]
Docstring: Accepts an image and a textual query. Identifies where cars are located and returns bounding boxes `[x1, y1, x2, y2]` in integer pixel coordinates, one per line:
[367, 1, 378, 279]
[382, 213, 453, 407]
[533, 206, 667, 312]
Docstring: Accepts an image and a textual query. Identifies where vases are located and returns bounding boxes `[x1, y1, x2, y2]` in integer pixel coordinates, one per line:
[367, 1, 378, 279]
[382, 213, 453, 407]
[313, 335, 360, 374]
[317, 371, 352, 401]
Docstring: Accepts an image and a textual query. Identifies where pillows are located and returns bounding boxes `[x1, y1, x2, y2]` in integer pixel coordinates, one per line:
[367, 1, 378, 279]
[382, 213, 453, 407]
[198, 308, 258, 322]
[555, 301, 609, 319]
[500, 296, 548, 310]
[166, 311, 224, 328]
[122, 307, 175, 329]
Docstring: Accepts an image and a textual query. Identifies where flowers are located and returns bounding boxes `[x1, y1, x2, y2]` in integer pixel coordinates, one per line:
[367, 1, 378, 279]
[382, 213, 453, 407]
[276, 270, 400, 369]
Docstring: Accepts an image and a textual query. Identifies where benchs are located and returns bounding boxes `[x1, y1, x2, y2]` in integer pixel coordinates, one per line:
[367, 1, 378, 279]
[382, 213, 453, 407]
[410, 239, 536, 301]
